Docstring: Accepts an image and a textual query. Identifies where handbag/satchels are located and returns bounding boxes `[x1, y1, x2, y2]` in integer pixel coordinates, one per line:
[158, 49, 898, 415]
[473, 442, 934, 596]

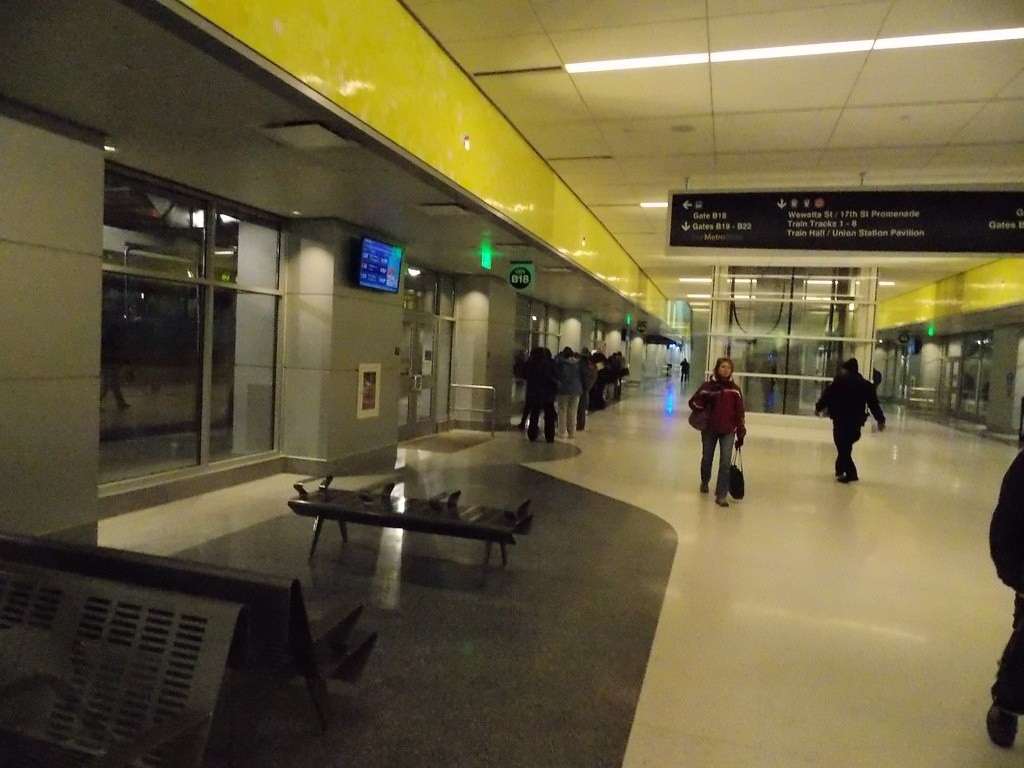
[688, 380, 716, 429]
[622, 367, 629, 376]
[728, 465, 744, 499]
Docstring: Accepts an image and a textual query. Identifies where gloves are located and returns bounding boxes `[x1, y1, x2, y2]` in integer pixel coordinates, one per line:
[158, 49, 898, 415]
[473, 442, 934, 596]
[734, 437, 744, 448]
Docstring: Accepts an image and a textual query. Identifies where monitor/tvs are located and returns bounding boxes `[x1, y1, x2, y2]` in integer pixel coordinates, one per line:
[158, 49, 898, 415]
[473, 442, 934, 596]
[356, 235, 403, 294]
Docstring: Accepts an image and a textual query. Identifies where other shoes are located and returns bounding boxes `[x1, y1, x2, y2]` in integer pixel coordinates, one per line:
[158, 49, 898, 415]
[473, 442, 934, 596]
[834, 463, 844, 475]
[838, 473, 858, 482]
[985, 696, 1020, 747]
[699, 480, 709, 493]
[715, 497, 729, 507]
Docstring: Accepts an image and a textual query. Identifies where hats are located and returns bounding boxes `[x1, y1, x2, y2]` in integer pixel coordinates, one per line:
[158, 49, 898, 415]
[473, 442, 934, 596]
[841, 358, 858, 373]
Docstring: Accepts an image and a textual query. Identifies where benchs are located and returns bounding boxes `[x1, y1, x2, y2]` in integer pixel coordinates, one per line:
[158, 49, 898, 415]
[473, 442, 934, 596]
[288, 469, 530, 594]
[0, 532, 382, 733]
[0, 554, 256, 768]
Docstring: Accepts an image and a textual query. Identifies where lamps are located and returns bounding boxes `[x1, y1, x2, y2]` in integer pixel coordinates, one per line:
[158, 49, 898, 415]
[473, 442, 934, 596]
[407, 264, 422, 278]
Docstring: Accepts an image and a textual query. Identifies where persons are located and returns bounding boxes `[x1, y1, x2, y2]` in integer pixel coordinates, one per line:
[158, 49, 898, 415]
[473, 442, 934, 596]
[814, 358, 886, 484]
[986, 447, 1024, 749]
[873, 367, 882, 404]
[964, 372, 974, 400]
[688, 357, 747, 507]
[101, 322, 130, 412]
[560, 345, 629, 440]
[680, 358, 689, 382]
[757, 349, 778, 413]
[513, 345, 559, 443]
